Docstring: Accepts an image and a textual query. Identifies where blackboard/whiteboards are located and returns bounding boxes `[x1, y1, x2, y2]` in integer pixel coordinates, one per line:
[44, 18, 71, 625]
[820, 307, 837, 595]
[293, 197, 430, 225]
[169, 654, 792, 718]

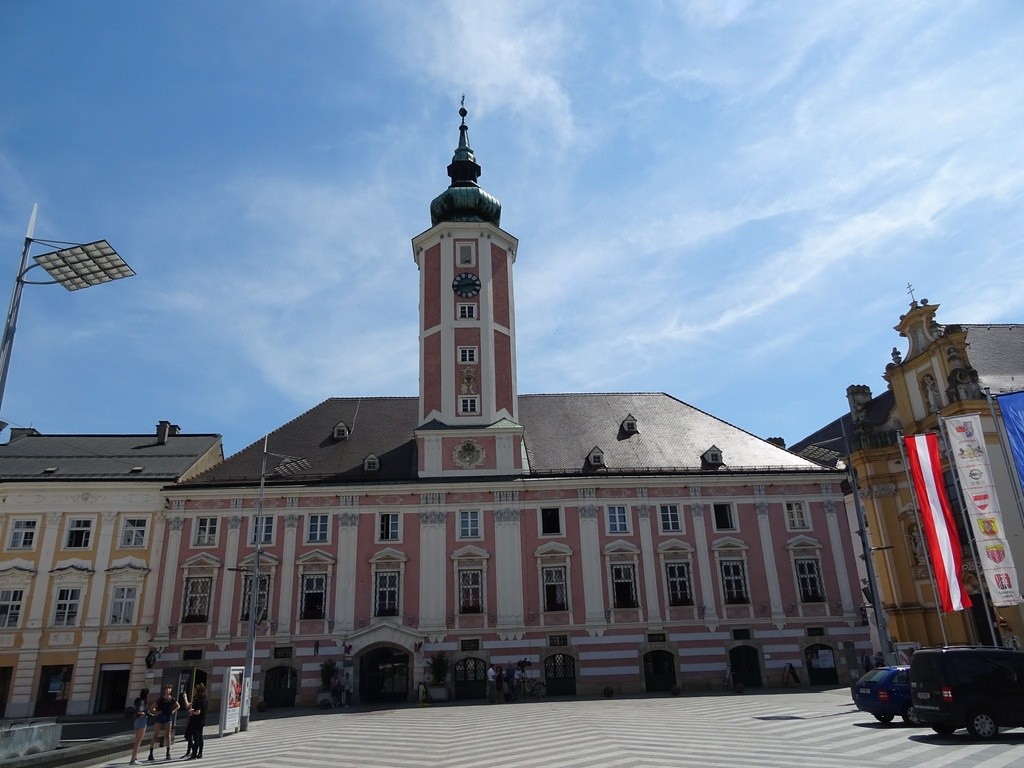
[782, 663, 802, 689]
[723, 664, 732, 687]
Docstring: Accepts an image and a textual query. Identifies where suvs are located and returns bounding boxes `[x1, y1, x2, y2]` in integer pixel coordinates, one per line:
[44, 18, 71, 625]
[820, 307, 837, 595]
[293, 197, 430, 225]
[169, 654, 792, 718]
[854, 666, 924, 727]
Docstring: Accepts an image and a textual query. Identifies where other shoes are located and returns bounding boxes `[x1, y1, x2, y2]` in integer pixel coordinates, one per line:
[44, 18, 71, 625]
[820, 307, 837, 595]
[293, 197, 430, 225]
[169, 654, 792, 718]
[129, 760, 142, 765]
[148, 755, 154, 761]
[189, 753, 202, 760]
[166, 753, 170, 760]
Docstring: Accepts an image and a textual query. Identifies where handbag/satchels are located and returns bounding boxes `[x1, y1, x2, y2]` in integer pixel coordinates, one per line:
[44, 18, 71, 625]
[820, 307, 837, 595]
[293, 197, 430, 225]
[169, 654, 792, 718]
[148, 705, 162, 717]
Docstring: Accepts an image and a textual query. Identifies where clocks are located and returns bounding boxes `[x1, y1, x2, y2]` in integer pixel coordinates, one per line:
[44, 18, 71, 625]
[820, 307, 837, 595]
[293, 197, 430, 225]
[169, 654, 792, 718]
[451, 271, 481, 299]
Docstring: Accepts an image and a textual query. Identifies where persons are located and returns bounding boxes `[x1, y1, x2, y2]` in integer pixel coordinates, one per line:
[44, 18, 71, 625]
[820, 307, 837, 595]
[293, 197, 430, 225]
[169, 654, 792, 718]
[180, 683, 208, 760]
[343, 672, 353, 708]
[148, 684, 181, 760]
[129, 688, 150, 765]
[862, 651, 874, 672]
[487, 664, 515, 703]
[812, 654, 819, 683]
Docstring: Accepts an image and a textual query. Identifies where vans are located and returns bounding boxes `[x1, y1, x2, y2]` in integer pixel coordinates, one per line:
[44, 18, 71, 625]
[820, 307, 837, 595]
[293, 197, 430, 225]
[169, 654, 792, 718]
[911, 642, 1024, 740]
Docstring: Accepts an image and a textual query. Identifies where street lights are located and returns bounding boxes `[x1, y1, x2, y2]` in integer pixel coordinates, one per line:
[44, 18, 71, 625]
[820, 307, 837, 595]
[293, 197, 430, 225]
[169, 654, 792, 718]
[1, 239, 137, 402]
[239, 457, 314, 729]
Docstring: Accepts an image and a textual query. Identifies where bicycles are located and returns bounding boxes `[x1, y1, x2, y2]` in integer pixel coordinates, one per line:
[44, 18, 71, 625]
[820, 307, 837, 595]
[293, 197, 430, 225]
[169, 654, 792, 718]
[514, 676, 543, 701]
